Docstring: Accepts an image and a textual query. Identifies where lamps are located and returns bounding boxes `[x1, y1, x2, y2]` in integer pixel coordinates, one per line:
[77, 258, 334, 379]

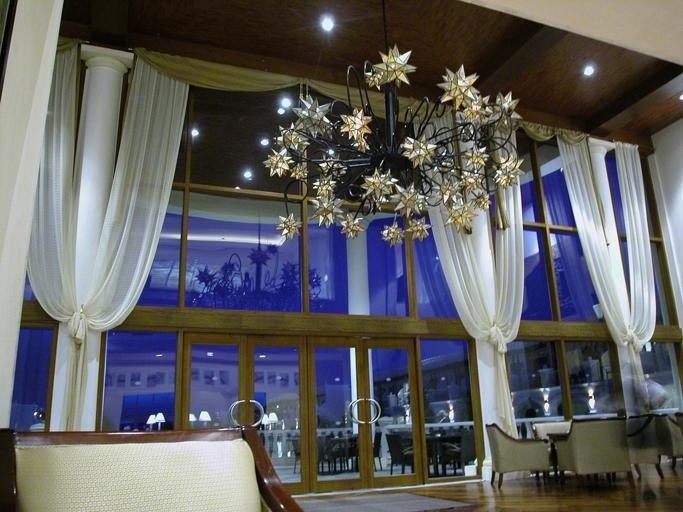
[264, 29, 522, 245]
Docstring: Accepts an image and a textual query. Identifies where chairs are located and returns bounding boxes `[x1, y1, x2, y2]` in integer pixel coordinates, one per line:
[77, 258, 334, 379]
[388, 428, 464, 477]
[486, 411, 683, 497]
[294, 428, 382, 474]
[0, 428, 304, 510]
